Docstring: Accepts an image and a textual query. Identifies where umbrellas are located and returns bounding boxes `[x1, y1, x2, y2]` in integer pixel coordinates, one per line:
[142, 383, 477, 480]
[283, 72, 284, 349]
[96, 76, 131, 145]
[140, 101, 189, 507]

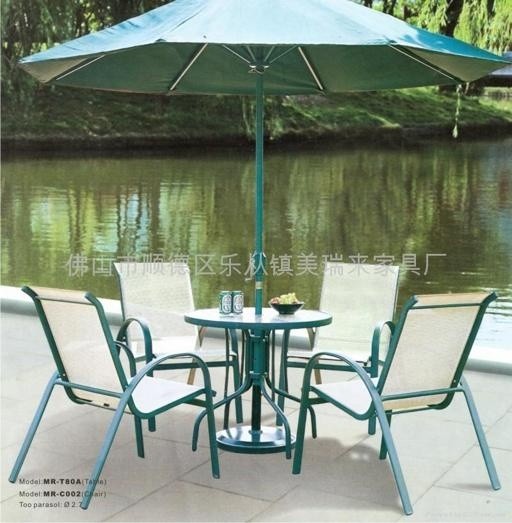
[17, 0, 512, 315]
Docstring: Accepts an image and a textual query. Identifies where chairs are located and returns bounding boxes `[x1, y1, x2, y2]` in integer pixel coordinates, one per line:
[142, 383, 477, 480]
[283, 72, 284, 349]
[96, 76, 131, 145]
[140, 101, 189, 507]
[7, 284, 220, 509]
[285, 260, 401, 437]
[292, 292, 502, 516]
[112, 259, 243, 432]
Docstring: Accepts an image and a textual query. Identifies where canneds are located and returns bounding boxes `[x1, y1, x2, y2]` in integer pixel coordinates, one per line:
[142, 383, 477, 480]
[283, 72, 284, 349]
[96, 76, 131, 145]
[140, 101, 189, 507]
[232, 291, 244, 313]
[218, 291, 232, 314]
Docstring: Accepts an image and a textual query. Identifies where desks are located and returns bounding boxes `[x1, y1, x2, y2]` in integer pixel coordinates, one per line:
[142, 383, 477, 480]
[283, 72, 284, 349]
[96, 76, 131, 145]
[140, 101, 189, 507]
[184, 305, 332, 453]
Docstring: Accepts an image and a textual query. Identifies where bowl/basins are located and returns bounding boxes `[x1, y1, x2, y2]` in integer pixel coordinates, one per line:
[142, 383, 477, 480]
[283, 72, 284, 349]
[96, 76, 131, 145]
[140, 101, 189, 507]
[271, 303, 303, 314]
